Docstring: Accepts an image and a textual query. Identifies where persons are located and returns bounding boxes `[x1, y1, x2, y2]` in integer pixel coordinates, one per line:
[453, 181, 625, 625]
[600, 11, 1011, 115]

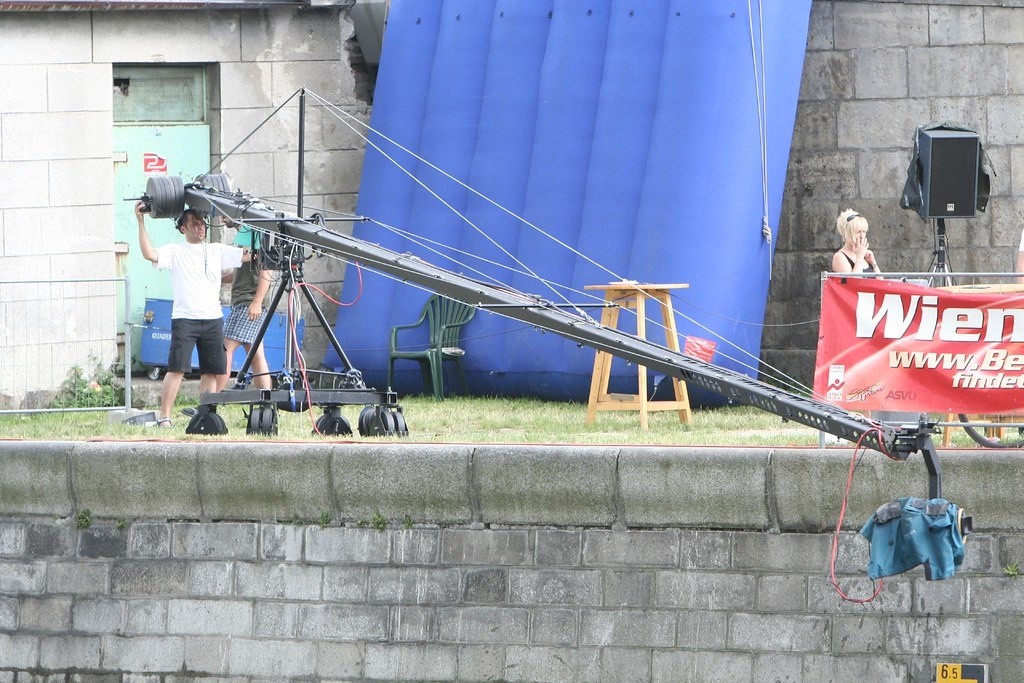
[180, 215, 280, 417]
[135, 200, 257, 429]
[1018, 227, 1024, 285]
[832, 208, 884, 280]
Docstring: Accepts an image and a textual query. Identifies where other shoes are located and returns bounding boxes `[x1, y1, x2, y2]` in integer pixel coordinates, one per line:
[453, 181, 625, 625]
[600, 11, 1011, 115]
[180, 407, 201, 416]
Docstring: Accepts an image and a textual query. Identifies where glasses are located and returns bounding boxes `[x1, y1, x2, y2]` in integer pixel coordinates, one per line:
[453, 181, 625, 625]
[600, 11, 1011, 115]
[844, 214, 864, 226]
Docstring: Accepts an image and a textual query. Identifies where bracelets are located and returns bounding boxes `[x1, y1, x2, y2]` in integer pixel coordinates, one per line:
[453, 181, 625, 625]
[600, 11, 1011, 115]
[870, 263, 877, 270]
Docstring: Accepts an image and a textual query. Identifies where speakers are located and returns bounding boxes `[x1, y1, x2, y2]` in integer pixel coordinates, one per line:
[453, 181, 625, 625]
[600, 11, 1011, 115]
[916, 128, 980, 217]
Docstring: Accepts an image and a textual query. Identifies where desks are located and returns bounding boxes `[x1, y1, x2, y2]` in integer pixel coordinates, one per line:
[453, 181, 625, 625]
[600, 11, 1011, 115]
[935, 284, 1024, 449]
[583, 283, 692, 432]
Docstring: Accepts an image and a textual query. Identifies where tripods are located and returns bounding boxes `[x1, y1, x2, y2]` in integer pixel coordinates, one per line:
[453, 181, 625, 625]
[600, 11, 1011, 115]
[913, 219, 956, 287]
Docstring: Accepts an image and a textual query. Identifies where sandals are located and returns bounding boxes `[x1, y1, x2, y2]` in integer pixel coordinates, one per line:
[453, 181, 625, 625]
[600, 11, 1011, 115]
[157, 418, 173, 429]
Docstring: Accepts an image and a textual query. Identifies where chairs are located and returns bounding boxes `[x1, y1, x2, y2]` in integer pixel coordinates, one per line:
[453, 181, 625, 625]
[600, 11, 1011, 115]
[387, 291, 471, 402]
